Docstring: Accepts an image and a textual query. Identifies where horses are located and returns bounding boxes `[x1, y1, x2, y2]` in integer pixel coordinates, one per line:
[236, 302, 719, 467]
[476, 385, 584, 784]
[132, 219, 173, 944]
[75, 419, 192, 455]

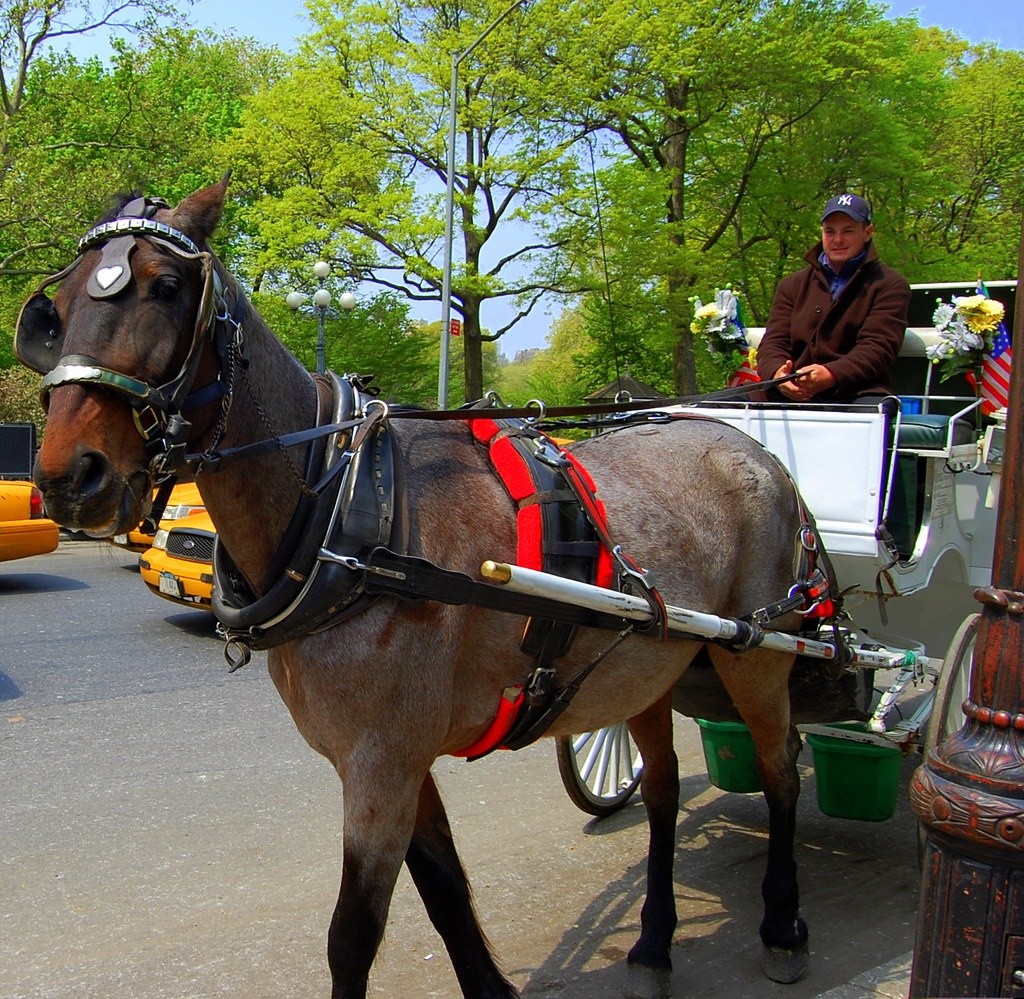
[33, 163, 826, 998]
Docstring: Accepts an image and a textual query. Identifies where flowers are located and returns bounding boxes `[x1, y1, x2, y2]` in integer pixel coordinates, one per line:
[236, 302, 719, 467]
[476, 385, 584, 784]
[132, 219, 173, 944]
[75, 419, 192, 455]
[686, 283, 758, 381]
[926, 295, 1006, 383]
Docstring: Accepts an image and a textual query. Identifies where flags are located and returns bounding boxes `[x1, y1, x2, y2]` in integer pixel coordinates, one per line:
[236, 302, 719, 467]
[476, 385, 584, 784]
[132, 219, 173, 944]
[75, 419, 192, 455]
[726, 297, 767, 402]
[964, 281, 1013, 413]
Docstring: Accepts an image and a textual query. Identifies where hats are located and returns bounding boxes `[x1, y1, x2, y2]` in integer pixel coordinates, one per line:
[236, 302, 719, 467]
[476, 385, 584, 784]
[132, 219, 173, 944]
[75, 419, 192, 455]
[820, 194, 870, 227]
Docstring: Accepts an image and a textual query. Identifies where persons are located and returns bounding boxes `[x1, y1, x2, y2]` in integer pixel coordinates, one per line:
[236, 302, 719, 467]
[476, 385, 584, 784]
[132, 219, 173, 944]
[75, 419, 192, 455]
[755, 194, 910, 547]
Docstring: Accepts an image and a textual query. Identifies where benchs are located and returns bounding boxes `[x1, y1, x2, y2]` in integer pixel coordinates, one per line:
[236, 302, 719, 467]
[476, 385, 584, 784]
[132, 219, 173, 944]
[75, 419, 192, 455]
[731, 328, 987, 458]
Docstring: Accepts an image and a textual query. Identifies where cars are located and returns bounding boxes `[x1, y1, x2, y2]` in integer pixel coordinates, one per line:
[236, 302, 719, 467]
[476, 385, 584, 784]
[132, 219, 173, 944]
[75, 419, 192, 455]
[0, 480, 59, 562]
[106, 482, 217, 612]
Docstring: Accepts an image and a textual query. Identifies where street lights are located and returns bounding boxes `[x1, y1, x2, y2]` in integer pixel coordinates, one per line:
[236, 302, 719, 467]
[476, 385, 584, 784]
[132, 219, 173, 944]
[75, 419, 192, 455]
[286, 261, 356, 376]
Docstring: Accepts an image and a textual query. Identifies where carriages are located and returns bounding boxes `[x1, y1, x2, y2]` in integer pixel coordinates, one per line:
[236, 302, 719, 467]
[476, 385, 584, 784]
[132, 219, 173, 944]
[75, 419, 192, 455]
[12, 136, 1016, 999]
